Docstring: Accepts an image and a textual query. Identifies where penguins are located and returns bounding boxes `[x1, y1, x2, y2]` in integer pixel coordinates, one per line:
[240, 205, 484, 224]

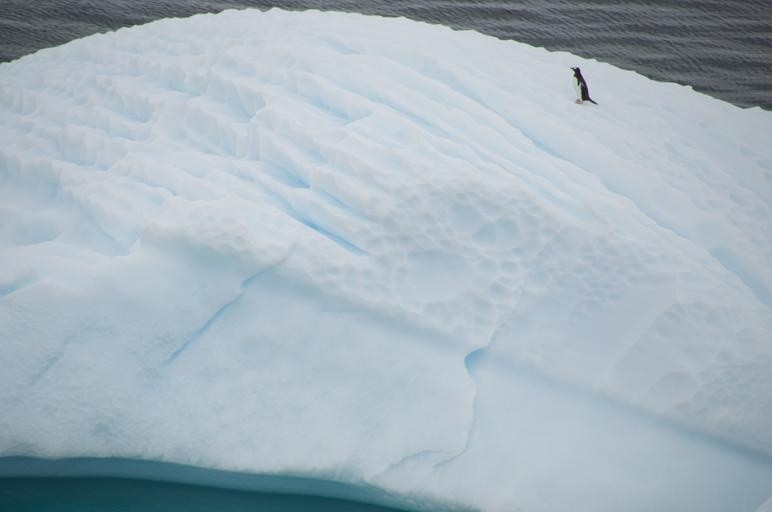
[569, 63, 599, 106]
